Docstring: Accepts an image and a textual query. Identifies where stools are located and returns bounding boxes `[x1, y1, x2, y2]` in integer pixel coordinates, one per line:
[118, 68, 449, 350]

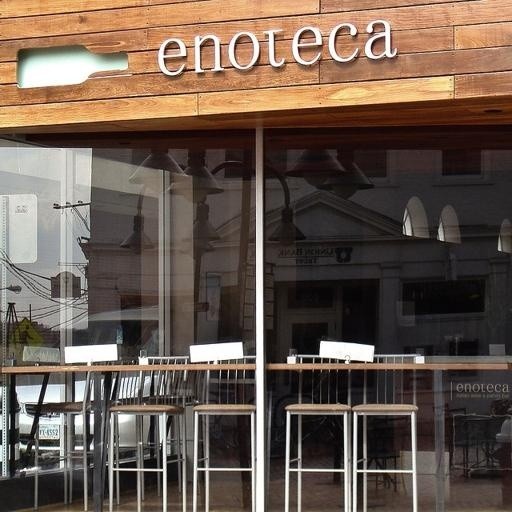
[106, 354, 188, 510]
[285, 354, 352, 511]
[1, 354, 133, 510]
[351, 355, 419, 511]
[192, 353, 273, 512]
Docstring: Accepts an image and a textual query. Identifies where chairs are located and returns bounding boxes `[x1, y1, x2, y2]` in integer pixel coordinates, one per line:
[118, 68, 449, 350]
[332, 397, 511, 494]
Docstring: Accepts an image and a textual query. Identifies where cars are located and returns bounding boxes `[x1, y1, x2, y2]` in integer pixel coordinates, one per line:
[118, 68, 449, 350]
[16, 375, 183, 469]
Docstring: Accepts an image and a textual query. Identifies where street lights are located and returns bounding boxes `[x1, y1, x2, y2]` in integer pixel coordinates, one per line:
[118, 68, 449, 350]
[116, 148, 309, 437]
[0, 285, 23, 295]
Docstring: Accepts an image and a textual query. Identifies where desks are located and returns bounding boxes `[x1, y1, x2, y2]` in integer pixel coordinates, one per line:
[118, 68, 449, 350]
[1, 363, 511, 511]
